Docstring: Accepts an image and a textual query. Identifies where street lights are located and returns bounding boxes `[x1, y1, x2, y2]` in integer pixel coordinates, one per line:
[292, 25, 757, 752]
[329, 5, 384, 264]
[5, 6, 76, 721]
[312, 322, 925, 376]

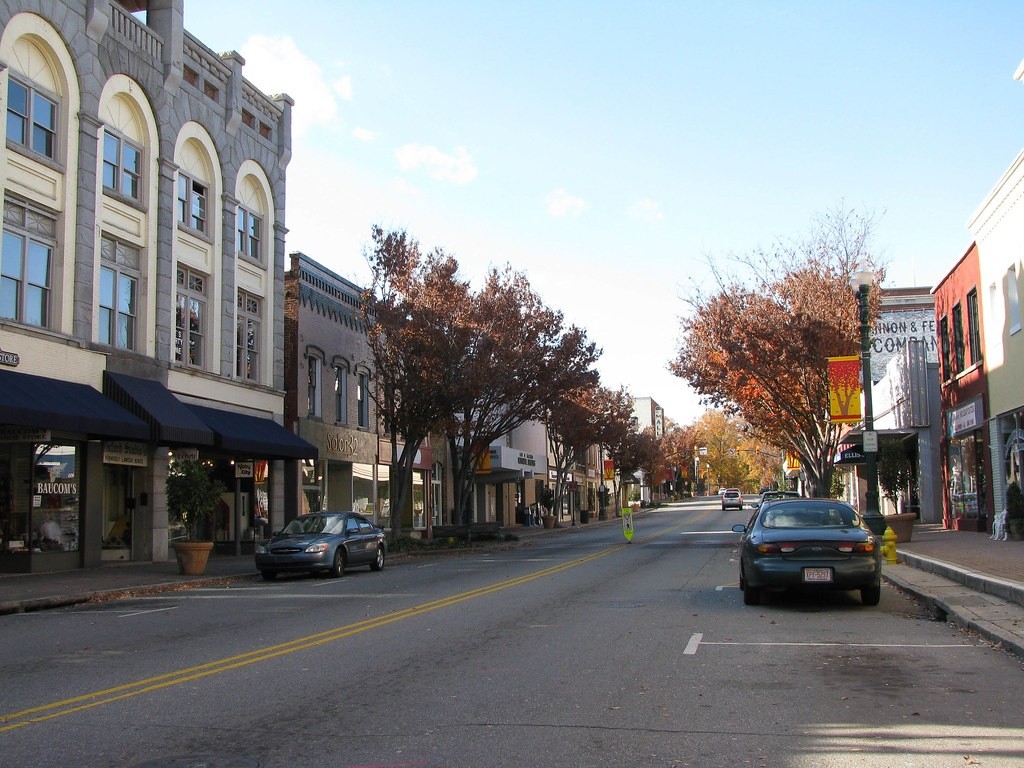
[596, 418, 608, 521]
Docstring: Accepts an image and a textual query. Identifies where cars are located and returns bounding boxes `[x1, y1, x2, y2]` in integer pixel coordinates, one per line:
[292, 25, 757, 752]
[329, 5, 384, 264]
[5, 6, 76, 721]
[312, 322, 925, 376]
[722, 488, 743, 511]
[718, 488, 726, 495]
[731, 498, 880, 606]
[751, 491, 806, 518]
[254, 512, 386, 582]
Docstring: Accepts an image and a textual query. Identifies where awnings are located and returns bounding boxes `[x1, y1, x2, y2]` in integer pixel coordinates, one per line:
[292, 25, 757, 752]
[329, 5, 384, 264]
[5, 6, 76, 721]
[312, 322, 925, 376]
[833, 444, 882, 465]
[0, 369, 149, 440]
[103, 369, 214, 447]
[181, 401, 318, 461]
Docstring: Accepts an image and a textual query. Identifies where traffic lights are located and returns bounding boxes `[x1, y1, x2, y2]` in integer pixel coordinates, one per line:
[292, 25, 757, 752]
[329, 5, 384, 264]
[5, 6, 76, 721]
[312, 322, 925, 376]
[756, 446, 760, 456]
[736, 446, 740, 456]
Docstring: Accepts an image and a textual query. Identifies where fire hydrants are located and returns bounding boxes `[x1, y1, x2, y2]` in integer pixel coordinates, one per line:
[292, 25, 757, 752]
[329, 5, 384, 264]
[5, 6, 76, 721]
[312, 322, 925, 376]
[881, 526, 898, 565]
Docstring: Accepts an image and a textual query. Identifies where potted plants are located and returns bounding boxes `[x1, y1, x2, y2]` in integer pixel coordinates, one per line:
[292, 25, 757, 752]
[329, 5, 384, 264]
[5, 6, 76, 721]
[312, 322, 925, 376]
[164, 456, 226, 575]
[876, 439, 917, 543]
[1006, 482, 1024, 542]
[540, 489, 557, 529]
[596, 488, 611, 520]
